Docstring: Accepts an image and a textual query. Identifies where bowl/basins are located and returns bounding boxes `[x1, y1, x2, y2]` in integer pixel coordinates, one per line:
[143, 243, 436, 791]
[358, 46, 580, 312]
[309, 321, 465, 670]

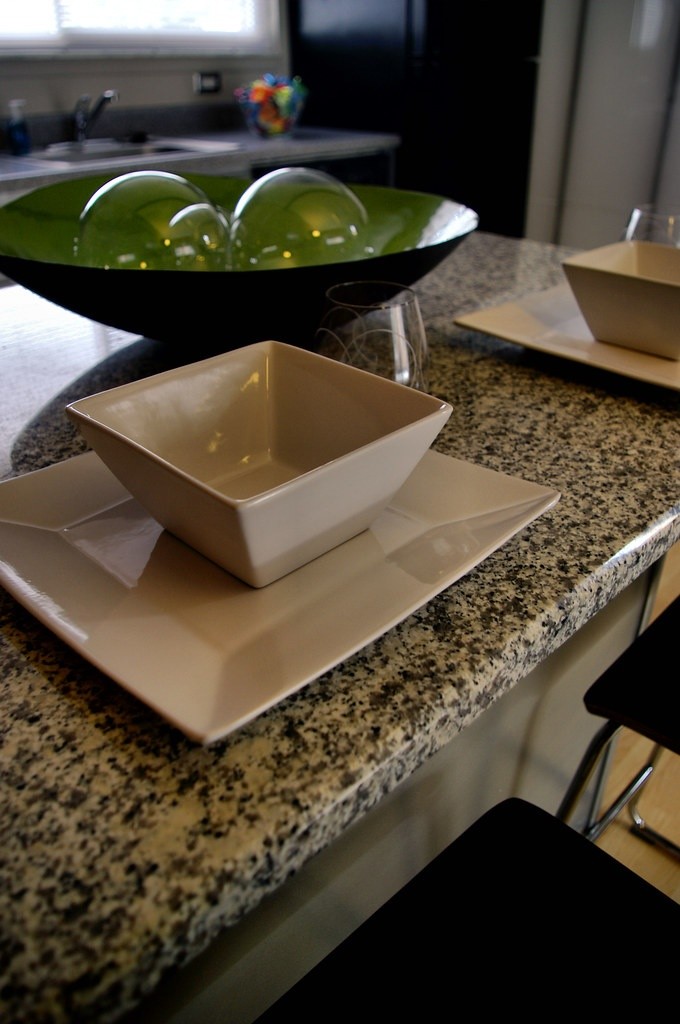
[563, 240, 680, 360]
[0, 170, 478, 354]
[66, 341, 454, 589]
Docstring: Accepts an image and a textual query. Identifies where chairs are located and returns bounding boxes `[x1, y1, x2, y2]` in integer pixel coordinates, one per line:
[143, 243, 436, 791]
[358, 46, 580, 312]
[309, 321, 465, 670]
[253, 797, 680, 1024]
[555, 594, 679, 867]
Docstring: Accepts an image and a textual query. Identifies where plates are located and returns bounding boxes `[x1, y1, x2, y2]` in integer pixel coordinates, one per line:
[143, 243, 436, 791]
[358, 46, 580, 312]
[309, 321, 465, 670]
[0, 449, 561, 746]
[455, 284, 680, 390]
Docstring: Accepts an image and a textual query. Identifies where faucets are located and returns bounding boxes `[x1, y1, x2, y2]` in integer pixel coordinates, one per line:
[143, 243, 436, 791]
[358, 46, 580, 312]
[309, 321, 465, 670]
[73, 90, 118, 153]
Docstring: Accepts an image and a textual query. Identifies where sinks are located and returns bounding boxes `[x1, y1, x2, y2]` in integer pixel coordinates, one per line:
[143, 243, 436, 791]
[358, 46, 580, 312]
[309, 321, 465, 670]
[2, 134, 245, 169]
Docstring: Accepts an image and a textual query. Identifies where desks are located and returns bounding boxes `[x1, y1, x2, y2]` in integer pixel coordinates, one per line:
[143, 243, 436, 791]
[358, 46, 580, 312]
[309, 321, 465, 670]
[0, 232, 679, 1024]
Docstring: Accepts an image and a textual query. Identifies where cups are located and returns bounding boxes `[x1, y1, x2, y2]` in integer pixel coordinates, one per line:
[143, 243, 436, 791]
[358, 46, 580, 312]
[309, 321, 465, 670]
[313, 281, 430, 396]
[623, 203, 680, 248]
